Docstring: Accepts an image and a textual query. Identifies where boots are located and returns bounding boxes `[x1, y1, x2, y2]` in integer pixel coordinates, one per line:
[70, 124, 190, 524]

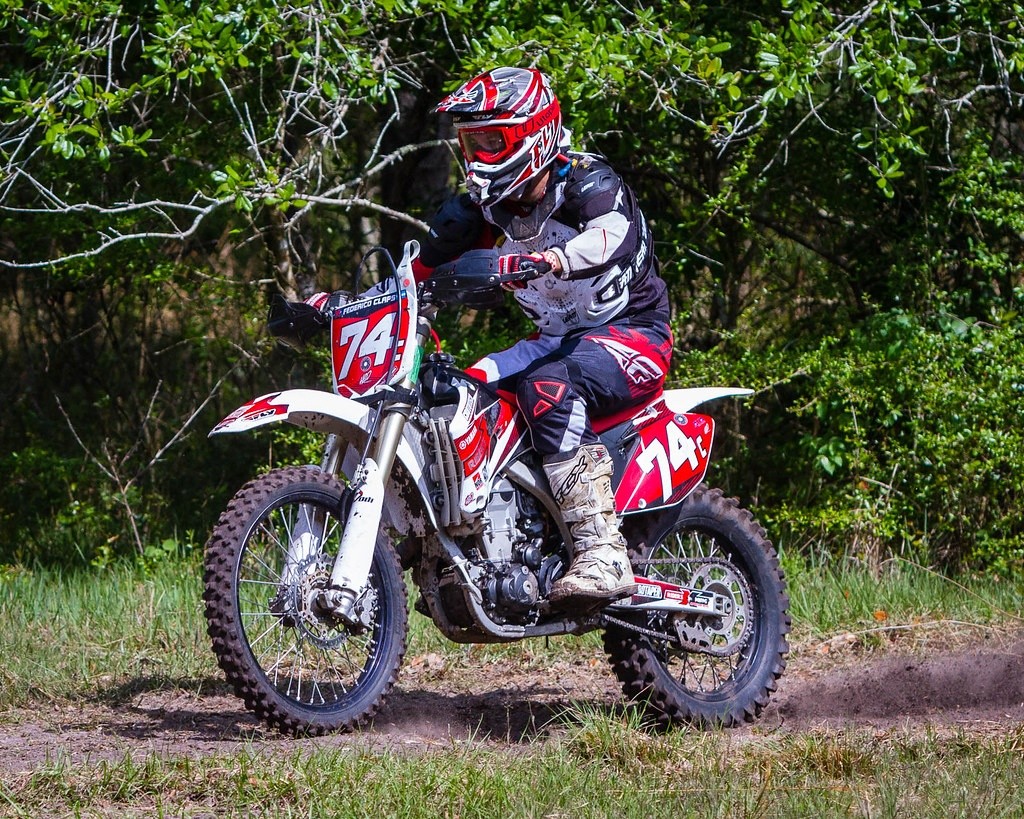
[544, 439, 640, 601]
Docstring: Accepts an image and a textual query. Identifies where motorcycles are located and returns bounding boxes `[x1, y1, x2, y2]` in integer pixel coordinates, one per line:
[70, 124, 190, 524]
[202, 240, 791, 740]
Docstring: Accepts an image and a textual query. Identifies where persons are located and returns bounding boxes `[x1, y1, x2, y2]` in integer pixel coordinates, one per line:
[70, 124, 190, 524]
[302, 66, 676, 618]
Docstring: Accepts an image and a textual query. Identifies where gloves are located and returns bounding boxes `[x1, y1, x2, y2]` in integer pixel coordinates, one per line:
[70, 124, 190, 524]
[299, 290, 334, 316]
[499, 244, 574, 292]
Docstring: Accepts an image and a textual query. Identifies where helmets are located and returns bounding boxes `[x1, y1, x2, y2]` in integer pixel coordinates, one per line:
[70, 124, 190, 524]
[427, 63, 570, 208]
